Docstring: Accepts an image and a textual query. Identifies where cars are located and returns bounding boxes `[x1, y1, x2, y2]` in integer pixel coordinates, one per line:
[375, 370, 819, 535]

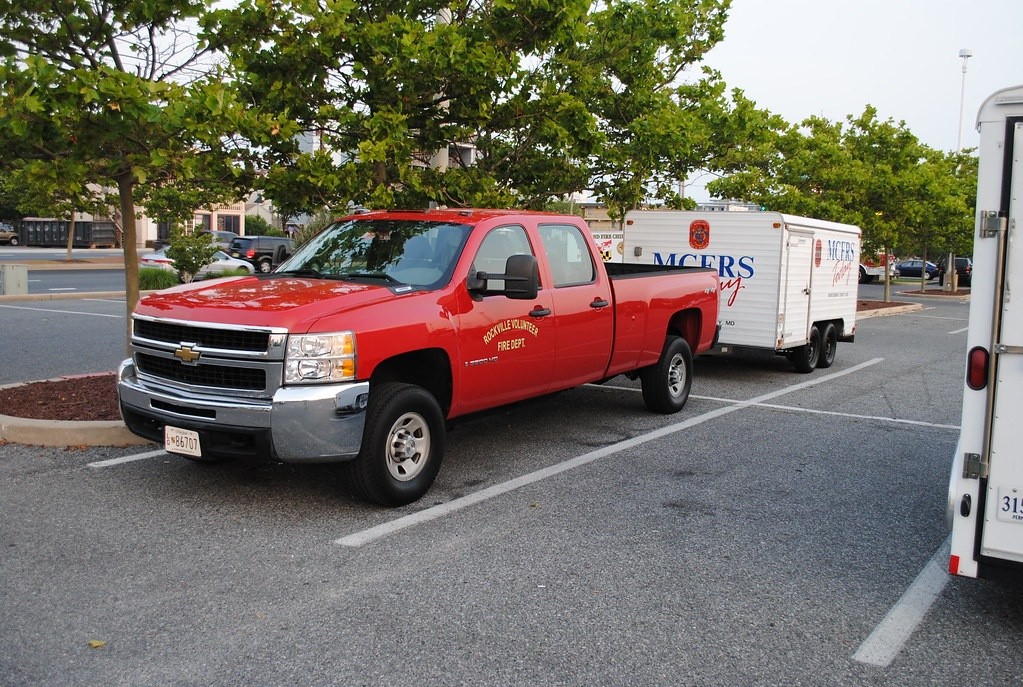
[0, 226, 20, 246]
[895, 260, 940, 280]
[138, 246, 255, 284]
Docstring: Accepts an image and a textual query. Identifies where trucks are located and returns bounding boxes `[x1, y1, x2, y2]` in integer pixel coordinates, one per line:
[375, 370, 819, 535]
[858, 247, 895, 283]
[947, 85, 1023, 578]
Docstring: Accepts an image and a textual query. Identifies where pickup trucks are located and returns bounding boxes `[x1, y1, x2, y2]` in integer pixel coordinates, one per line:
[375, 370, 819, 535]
[116, 206, 721, 507]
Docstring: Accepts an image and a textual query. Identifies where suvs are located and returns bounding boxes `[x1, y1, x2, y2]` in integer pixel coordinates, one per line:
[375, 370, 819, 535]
[196, 229, 298, 274]
[938, 258, 972, 287]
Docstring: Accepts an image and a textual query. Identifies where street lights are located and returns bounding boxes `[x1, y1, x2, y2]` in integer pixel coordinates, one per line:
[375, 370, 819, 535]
[941, 49, 972, 292]
